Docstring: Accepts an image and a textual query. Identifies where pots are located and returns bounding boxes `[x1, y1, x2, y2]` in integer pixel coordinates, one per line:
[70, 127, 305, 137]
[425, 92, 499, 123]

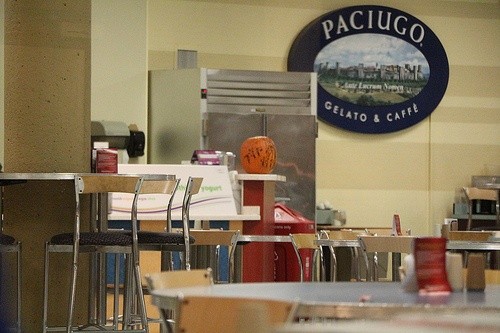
[317, 209, 338, 226]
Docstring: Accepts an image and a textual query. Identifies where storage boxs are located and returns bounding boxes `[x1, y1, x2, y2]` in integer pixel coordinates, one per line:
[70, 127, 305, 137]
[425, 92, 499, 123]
[95, 163, 239, 284]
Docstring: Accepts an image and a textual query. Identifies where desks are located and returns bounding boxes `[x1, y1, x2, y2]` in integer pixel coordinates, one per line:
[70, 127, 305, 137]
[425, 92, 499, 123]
[149, 281, 500, 333]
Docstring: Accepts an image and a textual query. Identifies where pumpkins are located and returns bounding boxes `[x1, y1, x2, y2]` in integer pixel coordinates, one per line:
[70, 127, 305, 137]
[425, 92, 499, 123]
[239, 136, 277, 174]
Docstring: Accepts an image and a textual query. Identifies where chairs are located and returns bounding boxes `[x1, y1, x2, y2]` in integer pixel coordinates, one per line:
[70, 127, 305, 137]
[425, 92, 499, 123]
[143, 267, 214, 333]
[113, 173, 204, 333]
[42, 174, 149, 333]
[0, 233, 22, 333]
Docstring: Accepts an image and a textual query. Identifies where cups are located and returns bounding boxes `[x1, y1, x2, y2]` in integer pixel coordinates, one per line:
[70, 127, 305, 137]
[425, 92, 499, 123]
[465, 254, 486, 292]
[215, 150, 236, 171]
[444, 252, 465, 293]
